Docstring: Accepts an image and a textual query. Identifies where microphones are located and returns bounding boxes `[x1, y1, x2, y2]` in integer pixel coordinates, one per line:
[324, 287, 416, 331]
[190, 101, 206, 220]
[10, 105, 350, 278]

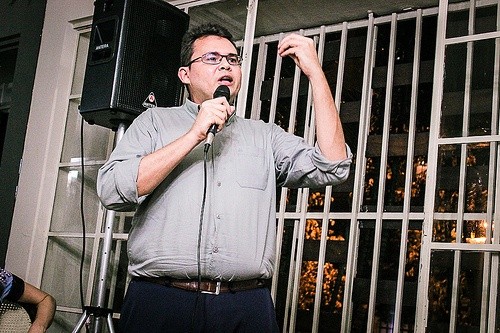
[204, 86, 229, 153]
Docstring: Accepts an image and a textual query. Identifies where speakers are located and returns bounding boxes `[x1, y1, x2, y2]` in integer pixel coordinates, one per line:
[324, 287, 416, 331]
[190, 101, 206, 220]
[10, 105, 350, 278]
[79, 0, 189, 133]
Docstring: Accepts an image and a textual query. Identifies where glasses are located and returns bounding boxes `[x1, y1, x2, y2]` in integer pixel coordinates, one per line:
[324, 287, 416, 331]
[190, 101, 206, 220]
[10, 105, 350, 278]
[184, 51, 243, 66]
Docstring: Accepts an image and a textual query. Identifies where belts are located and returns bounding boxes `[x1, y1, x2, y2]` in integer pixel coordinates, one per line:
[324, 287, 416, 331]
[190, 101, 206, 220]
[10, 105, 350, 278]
[133, 274, 270, 294]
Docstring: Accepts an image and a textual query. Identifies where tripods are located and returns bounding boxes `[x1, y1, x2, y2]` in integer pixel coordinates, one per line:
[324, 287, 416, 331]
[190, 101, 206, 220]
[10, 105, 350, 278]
[69, 123, 126, 333]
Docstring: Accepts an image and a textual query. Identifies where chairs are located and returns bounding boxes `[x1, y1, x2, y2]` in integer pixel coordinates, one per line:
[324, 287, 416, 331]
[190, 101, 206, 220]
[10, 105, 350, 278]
[0, 299, 37, 333]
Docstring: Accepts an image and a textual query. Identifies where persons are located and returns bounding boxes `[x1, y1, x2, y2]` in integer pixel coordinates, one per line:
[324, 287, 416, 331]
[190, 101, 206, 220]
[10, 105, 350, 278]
[0, 267, 56, 333]
[97, 22, 356, 333]
